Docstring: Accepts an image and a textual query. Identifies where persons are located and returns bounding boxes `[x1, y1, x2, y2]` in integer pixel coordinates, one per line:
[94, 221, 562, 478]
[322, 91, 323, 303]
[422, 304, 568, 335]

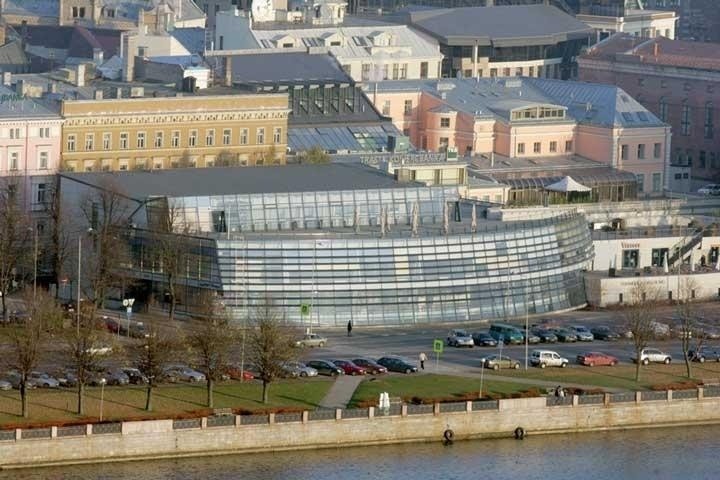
[559, 389, 564, 404]
[347, 320, 353, 337]
[418, 351, 428, 370]
[554, 384, 560, 404]
